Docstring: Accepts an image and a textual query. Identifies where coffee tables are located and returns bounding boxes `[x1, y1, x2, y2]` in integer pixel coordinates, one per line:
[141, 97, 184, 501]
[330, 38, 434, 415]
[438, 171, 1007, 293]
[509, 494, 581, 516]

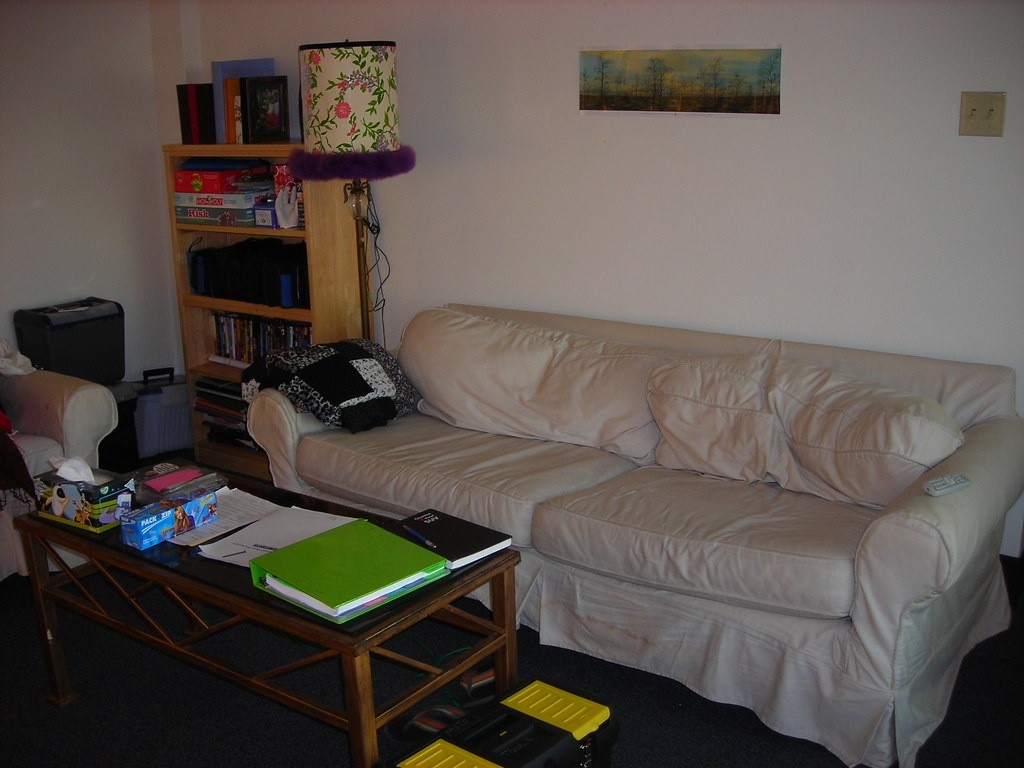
[13, 460, 521, 767]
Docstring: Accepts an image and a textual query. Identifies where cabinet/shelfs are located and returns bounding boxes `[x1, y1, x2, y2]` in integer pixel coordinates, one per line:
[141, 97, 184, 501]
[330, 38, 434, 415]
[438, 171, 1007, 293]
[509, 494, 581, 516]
[159, 140, 374, 483]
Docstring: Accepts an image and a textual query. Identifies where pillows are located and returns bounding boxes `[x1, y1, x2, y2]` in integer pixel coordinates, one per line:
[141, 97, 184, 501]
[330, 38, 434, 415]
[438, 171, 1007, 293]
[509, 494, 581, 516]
[640, 355, 970, 510]
[393, 306, 673, 466]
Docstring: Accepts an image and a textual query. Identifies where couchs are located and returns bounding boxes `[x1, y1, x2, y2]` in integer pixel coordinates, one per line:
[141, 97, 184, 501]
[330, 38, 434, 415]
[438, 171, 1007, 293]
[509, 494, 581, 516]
[250, 306, 1023, 768]
[0, 365, 120, 585]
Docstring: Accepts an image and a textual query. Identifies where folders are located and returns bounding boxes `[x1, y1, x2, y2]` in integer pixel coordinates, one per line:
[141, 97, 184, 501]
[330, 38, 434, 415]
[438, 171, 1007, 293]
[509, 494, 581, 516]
[248, 519, 450, 624]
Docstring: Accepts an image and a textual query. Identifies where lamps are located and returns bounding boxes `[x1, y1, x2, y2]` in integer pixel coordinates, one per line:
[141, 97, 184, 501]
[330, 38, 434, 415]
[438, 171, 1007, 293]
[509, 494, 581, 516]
[288, 38, 417, 343]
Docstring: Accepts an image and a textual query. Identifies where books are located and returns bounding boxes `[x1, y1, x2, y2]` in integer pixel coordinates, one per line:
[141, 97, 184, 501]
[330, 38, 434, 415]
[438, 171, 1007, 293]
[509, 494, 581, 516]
[240, 76, 290, 144]
[192, 377, 250, 425]
[385, 507, 513, 571]
[213, 312, 312, 364]
[211, 58, 274, 144]
[125, 457, 227, 495]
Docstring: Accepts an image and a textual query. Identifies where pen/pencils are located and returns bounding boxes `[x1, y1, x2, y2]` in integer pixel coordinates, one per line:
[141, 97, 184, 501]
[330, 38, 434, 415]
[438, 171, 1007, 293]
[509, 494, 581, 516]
[402, 525, 437, 549]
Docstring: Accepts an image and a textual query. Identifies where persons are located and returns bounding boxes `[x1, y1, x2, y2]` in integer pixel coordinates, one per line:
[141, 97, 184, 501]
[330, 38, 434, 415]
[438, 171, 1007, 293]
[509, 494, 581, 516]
[259, 102, 281, 130]
[171, 506, 195, 538]
[275, 186, 299, 229]
